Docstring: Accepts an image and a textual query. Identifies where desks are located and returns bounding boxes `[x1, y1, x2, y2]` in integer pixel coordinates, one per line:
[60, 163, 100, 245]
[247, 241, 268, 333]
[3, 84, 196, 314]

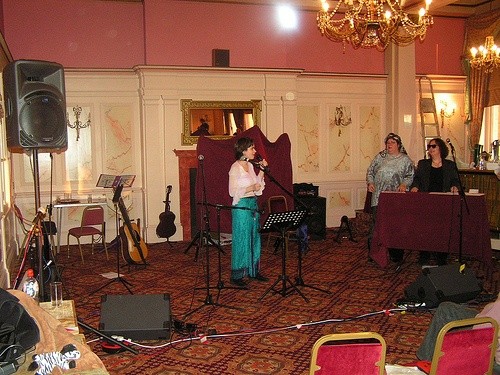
[37, 300, 78, 336]
[369, 189, 491, 277]
[458, 168, 500, 233]
[53, 203, 107, 253]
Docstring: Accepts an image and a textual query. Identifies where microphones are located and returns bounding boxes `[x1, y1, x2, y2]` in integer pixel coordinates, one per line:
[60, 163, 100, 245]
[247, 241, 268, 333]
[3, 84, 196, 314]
[446, 138, 456, 152]
[256, 154, 271, 172]
[197, 154, 204, 170]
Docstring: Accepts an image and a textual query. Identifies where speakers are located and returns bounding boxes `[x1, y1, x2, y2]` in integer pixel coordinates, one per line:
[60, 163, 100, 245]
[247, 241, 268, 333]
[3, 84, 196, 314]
[3, 59, 69, 154]
[402, 265, 481, 310]
[293, 195, 327, 242]
[99, 292, 171, 340]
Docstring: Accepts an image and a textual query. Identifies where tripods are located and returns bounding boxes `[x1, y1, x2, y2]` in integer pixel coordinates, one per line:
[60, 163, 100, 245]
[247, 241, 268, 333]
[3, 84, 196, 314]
[258, 211, 310, 305]
[12, 149, 70, 301]
[181, 164, 259, 319]
[264, 167, 332, 298]
[85, 174, 136, 298]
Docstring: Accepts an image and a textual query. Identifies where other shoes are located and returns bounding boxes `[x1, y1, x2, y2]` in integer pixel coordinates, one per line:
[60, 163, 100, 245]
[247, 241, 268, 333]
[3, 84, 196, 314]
[418, 258, 429, 265]
[437, 258, 448, 266]
[402, 360, 432, 374]
[248, 273, 269, 281]
[230, 277, 249, 289]
[391, 256, 403, 263]
[368, 258, 375, 262]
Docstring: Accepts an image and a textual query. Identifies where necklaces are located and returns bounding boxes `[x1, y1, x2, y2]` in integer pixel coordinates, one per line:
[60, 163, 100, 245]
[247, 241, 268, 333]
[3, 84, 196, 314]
[433, 161, 441, 166]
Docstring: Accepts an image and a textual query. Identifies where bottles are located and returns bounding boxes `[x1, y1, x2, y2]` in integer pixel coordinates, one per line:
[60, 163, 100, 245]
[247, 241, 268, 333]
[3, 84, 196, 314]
[22, 269, 40, 307]
[87, 193, 92, 204]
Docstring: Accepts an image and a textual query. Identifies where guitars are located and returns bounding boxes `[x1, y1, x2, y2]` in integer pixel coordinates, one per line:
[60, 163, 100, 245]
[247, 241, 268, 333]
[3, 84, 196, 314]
[112, 185, 149, 264]
[155, 185, 176, 239]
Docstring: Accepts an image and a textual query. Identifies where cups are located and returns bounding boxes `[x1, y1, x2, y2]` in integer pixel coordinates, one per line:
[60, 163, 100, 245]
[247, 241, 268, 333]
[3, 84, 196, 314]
[49, 281, 63, 306]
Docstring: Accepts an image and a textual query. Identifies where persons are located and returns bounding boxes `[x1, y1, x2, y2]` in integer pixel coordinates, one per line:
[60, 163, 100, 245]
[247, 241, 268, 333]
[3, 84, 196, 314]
[416, 292, 500, 365]
[229, 138, 269, 285]
[409, 138, 458, 264]
[366, 134, 415, 262]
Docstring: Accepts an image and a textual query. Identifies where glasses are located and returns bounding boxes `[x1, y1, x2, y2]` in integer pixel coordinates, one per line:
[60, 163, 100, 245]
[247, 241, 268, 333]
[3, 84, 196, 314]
[427, 144, 439, 148]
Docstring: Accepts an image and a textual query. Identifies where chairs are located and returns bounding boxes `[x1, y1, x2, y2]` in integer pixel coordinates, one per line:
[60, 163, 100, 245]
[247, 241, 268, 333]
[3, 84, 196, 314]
[268, 195, 311, 259]
[309, 332, 386, 375]
[406, 318, 499, 375]
[67, 206, 109, 264]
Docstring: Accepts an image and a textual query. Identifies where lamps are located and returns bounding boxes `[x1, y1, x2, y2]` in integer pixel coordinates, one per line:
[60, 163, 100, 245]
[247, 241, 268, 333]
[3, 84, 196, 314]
[469, 44, 500, 73]
[315, 0, 434, 52]
